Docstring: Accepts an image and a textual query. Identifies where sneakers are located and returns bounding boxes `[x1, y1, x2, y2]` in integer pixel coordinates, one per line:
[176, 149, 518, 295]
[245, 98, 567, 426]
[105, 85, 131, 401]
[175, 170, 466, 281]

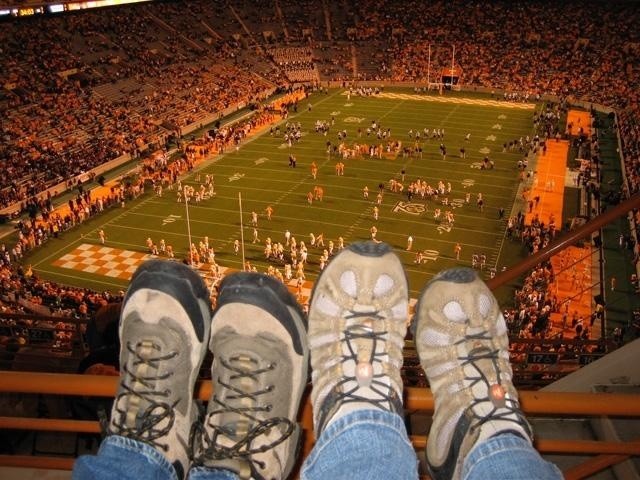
[189, 272, 308, 480]
[307, 241, 408, 442]
[106, 258, 212, 480]
[413, 266, 534, 479]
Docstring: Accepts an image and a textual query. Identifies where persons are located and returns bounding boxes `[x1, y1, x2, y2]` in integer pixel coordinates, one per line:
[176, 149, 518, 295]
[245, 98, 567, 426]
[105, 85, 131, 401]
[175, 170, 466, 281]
[0, 0, 640, 480]
